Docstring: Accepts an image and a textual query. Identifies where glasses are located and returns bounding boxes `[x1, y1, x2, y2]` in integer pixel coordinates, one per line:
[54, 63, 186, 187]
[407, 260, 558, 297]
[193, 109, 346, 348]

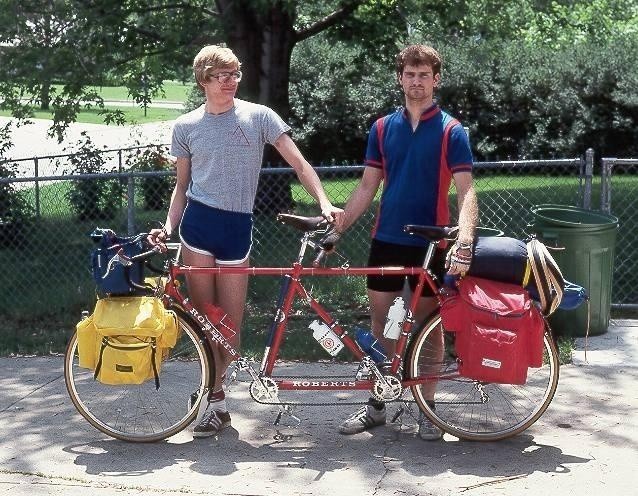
[210, 70, 243, 84]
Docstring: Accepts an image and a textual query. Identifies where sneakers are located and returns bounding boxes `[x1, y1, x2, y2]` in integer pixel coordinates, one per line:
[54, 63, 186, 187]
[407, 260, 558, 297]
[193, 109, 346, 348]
[192, 408, 233, 438]
[185, 372, 227, 411]
[418, 406, 443, 441]
[338, 401, 388, 436]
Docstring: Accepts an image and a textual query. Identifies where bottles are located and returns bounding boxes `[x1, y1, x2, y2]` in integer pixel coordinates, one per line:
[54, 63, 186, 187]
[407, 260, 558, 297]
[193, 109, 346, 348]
[383, 296, 407, 341]
[351, 325, 388, 363]
[307, 319, 345, 357]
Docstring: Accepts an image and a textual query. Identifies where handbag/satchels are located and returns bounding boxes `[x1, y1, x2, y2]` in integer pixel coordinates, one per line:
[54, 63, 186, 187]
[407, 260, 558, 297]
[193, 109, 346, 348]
[440, 275, 544, 388]
[73, 294, 179, 389]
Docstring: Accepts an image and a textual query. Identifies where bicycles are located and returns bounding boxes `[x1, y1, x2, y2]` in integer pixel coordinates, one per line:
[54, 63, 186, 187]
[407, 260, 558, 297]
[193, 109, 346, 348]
[59, 213, 560, 447]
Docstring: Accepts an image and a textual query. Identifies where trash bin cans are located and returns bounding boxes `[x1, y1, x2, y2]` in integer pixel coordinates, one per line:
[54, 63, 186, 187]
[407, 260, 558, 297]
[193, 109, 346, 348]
[527, 204, 620, 338]
[444, 227, 505, 358]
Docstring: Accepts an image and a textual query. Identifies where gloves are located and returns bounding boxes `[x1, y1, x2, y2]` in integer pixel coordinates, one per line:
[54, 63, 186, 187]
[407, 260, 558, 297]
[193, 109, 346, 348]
[440, 240, 476, 279]
[314, 226, 342, 254]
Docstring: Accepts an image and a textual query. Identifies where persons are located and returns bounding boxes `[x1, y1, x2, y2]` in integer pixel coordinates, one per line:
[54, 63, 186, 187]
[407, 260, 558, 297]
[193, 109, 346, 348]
[318, 41, 480, 442]
[142, 43, 347, 439]
